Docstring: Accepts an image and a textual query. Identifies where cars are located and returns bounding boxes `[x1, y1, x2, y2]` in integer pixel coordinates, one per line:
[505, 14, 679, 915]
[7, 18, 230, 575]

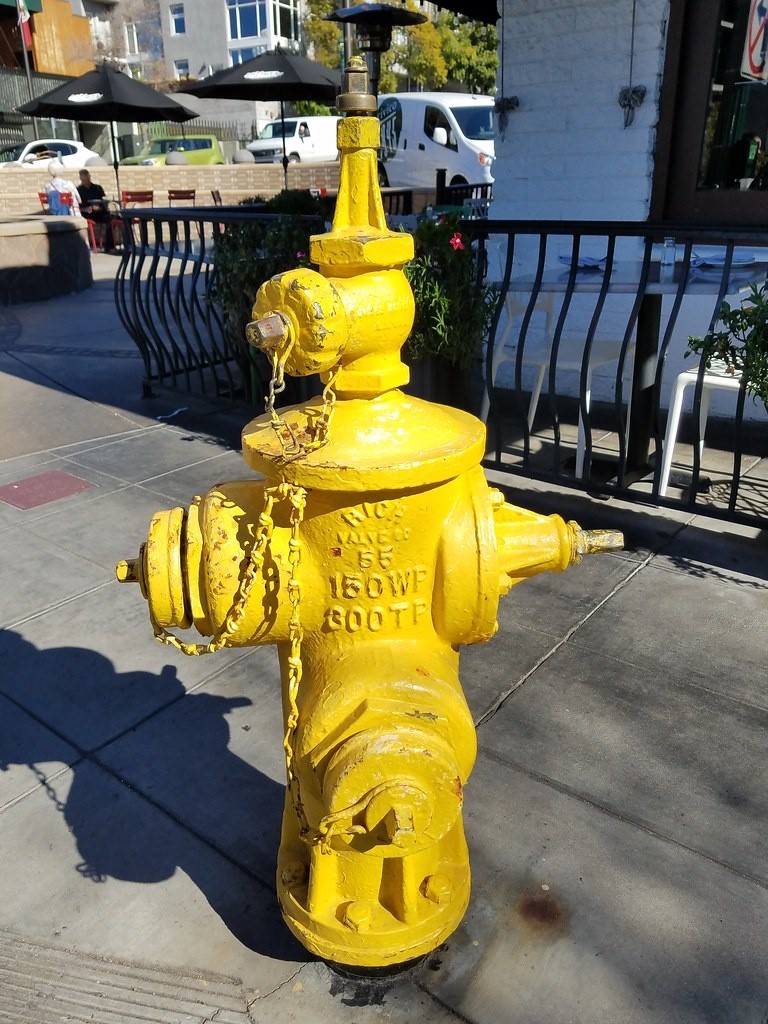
[0, 139, 100, 167]
[119, 134, 227, 166]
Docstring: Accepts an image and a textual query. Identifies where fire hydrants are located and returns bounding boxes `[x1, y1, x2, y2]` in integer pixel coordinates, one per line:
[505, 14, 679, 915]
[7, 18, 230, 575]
[113, 53, 630, 968]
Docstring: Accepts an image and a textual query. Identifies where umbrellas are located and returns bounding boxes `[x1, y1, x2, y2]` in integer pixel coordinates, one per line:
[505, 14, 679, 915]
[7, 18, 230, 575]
[14, 57, 201, 220]
[175, 39, 352, 200]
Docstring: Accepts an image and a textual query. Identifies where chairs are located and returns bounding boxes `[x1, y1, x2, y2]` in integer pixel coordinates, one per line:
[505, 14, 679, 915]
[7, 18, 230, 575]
[210, 189, 227, 234]
[37, 191, 98, 253]
[110, 191, 155, 254]
[166, 189, 201, 243]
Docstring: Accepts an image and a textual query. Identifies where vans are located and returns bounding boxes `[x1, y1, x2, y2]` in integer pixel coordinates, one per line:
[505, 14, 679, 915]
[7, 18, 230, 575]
[247, 116, 343, 164]
[376, 91, 494, 199]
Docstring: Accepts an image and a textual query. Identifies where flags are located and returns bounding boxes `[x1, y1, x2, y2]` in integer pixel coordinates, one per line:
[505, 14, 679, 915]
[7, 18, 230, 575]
[17, 0, 34, 51]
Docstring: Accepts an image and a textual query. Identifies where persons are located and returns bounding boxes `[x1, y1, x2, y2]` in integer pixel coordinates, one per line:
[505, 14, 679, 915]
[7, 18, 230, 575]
[74, 169, 122, 256]
[44, 159, 86, 221]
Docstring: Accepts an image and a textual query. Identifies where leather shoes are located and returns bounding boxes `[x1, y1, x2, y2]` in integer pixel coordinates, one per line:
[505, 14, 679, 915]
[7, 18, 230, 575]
[106, 248, 121, 255]
